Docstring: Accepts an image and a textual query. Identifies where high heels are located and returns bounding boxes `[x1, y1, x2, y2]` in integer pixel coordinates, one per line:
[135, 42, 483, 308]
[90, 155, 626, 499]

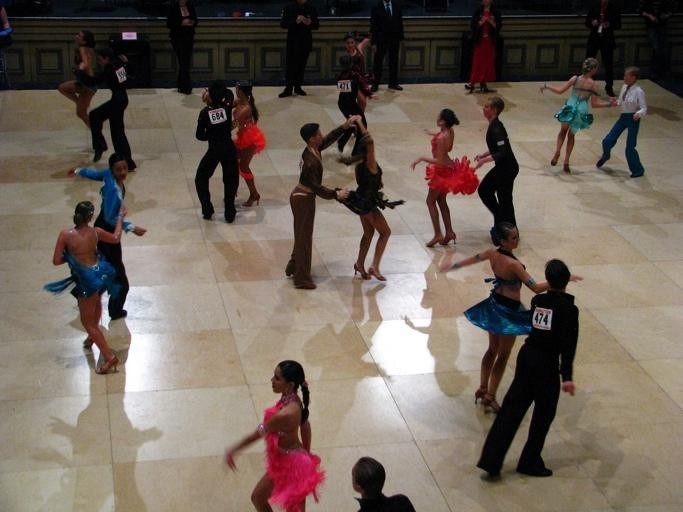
[440, 233, 457, 245]
[465, 84, 475, 94]
[95, 355, 119, 372]
[563, 163, 570, 174]
[242, 193, 260, 207]
[368, 267, 387, 281]
[474, 384, 488, 403]
[481, 393, 500, 414]
[425, 234, 443, 248]
[83, 338, 94, 348]
[550, 152, 559, 166]
[480, 84, 490, 92]
[353, 263, 371, 280]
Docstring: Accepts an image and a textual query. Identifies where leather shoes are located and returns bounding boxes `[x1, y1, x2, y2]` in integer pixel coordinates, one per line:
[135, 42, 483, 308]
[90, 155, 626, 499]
[294, 282, 316, 289]
[202, 210, 214, 220]
[596, 156, 610, 167]
[111, 310, 128, 318]
[517, 467, 552, 477]
[607, 90, 615, 96]
[338, 138, 343, 151]
[630, 172, 645, 178]
[476, 461, 499, 476]
[93, 144, 108, 162]
[226, 210, 236, 221]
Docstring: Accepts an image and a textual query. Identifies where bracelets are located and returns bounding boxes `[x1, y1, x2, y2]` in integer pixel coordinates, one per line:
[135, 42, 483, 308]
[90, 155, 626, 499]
[608, 101, 611, 106]
[544, 84, 548, 89]
[454, 262, 459, 268]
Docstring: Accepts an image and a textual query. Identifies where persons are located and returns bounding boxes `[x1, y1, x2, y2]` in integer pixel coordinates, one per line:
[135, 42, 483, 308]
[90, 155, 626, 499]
[474, 97, 519, 225]
[476, 259, 581, 476]
[411, 109, 479, 247]
[596, 66, 647, 177]
[441, 222, 584, 414]
[225, 361, 326, 512]
[539, 58, 620, 172]
[45, 29, 146, 374]
[465, 0, 502, 94]
[639, 0, 672, 81]
[585, 0, 622, 97]
[0, 1, 13, 90]
[352, 458, 416, 512]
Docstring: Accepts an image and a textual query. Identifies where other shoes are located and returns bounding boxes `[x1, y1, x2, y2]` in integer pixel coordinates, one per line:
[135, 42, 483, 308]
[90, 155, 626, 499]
[370, 85, 379, 92]
[278, 88, 292, 98]
[178, 87, 192, 94]
[294, 86, 306, 96]
[388, 84, 403, 90]
